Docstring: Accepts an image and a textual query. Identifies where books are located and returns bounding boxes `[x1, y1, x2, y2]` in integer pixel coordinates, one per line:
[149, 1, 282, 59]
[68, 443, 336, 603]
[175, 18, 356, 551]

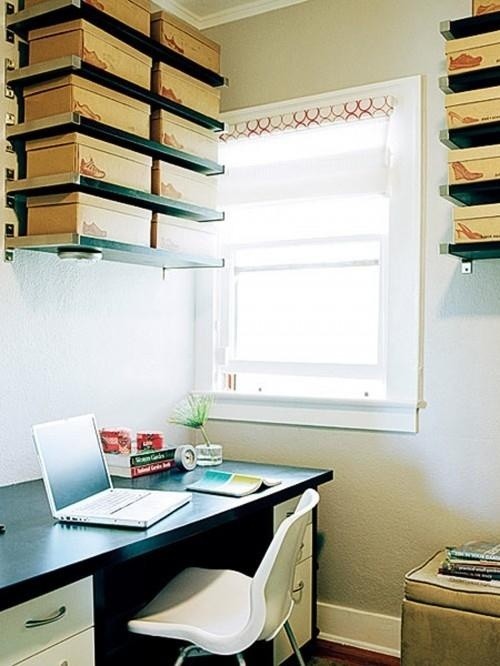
[186, 469, 284, 499]
[103, 446, 178, 466]
[436, 539, 500, 587]
[106, 459, 179, 478]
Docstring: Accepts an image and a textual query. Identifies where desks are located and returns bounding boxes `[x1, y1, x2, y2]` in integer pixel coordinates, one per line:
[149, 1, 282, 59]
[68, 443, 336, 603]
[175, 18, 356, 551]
[0, 457, 334, 665]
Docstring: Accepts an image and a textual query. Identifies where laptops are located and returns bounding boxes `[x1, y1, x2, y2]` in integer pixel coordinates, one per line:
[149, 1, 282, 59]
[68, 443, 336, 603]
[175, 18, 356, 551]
[31, 412, 193, 530]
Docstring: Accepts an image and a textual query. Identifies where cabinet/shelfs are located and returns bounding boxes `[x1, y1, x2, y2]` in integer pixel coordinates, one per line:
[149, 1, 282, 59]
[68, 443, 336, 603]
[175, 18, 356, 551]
[444, 1, 500, 245]
[2, 569, 98, 664]
[437, 9, 500, 260]
[1, 0, 227, 272]
